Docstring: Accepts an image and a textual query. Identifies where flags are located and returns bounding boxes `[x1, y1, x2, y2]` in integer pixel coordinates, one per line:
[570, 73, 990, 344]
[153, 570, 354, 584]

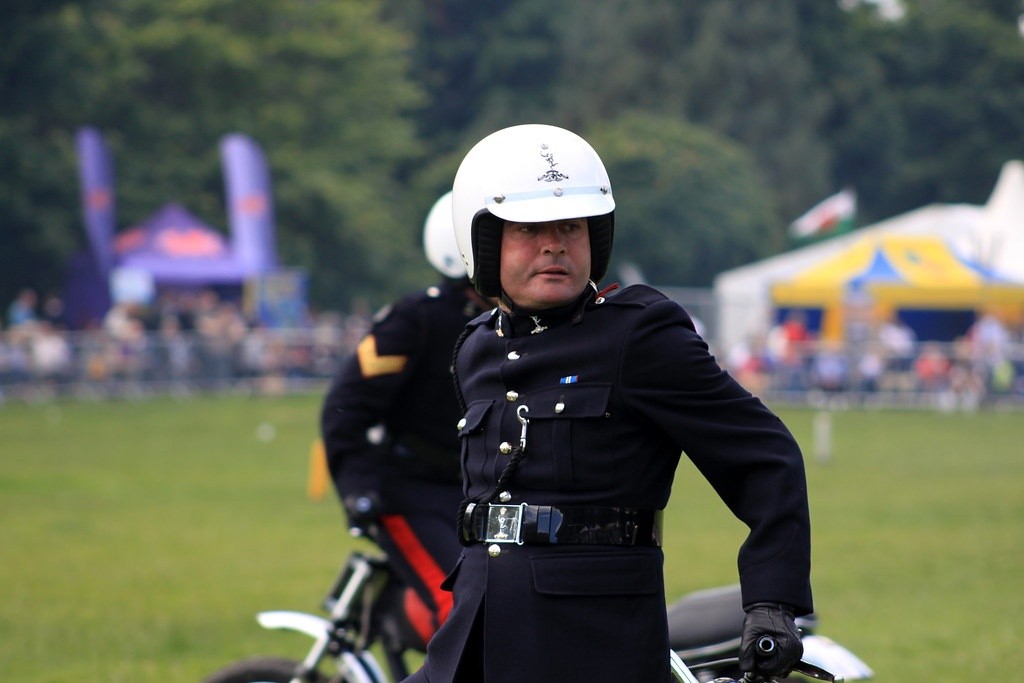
[792, 192, 857, 245]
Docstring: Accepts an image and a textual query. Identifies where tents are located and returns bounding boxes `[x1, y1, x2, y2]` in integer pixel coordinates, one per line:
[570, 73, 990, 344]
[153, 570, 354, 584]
[722, 161, 1024, 393]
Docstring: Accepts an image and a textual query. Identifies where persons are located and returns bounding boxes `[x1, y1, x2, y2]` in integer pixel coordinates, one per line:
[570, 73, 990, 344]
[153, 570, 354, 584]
[317, 188, 487, 662]
[0, 294, 1024, 415]
[402, 123, 814, 683]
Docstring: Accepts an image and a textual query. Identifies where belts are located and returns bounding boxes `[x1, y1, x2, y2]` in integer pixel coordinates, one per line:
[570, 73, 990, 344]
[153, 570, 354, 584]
[455, 500, 655, 548]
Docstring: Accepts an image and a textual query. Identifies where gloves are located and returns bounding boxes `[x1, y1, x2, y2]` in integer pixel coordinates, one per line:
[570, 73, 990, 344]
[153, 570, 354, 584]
[345, 478, 385, 528]
[737, 603, 804, 679]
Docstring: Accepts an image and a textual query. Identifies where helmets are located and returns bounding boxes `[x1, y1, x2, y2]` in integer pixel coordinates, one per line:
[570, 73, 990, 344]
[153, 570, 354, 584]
[453, 124, 616, 280]
[422, 189, 468, 281]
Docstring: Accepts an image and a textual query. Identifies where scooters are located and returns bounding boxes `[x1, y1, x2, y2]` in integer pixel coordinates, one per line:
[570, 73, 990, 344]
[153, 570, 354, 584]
[202, 521, 877, 683]
